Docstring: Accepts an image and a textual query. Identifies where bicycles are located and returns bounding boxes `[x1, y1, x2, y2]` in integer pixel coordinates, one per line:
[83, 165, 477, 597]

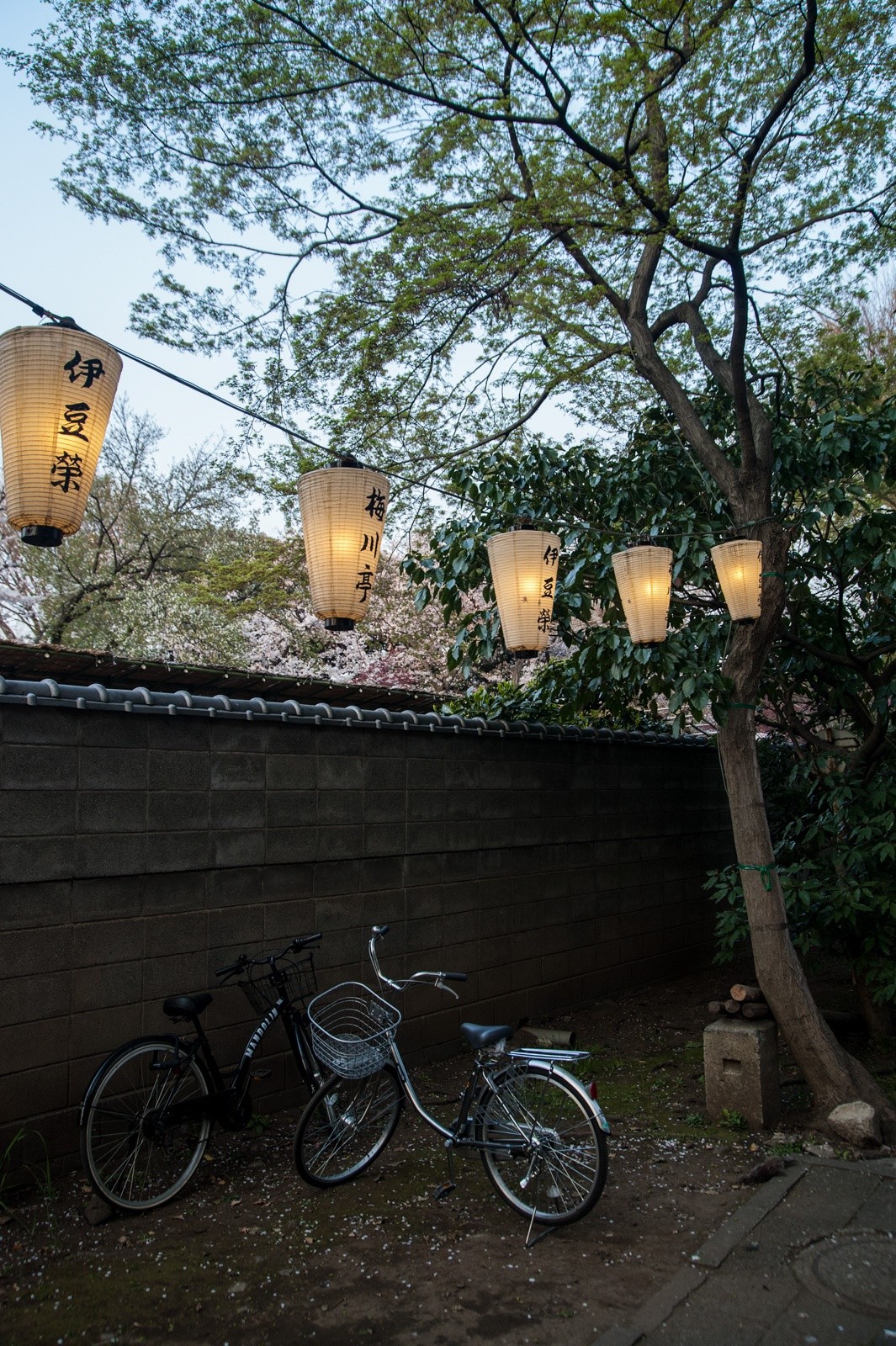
[289, 923, 614, 1249]
[77, 932, 352, 1218]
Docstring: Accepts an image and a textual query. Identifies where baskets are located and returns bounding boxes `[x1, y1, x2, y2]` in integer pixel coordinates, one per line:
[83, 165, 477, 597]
[308, 982, 402, 1079]
[238, 953, 317, 1016]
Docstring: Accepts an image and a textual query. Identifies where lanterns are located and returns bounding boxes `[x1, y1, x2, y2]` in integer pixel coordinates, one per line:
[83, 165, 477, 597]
[710, 536, 762, 626]
[610, 541, 673, 649]
[0, 317, 123, 547]
[486, 526, 560, 658]
[296, 453, 390, 630]
